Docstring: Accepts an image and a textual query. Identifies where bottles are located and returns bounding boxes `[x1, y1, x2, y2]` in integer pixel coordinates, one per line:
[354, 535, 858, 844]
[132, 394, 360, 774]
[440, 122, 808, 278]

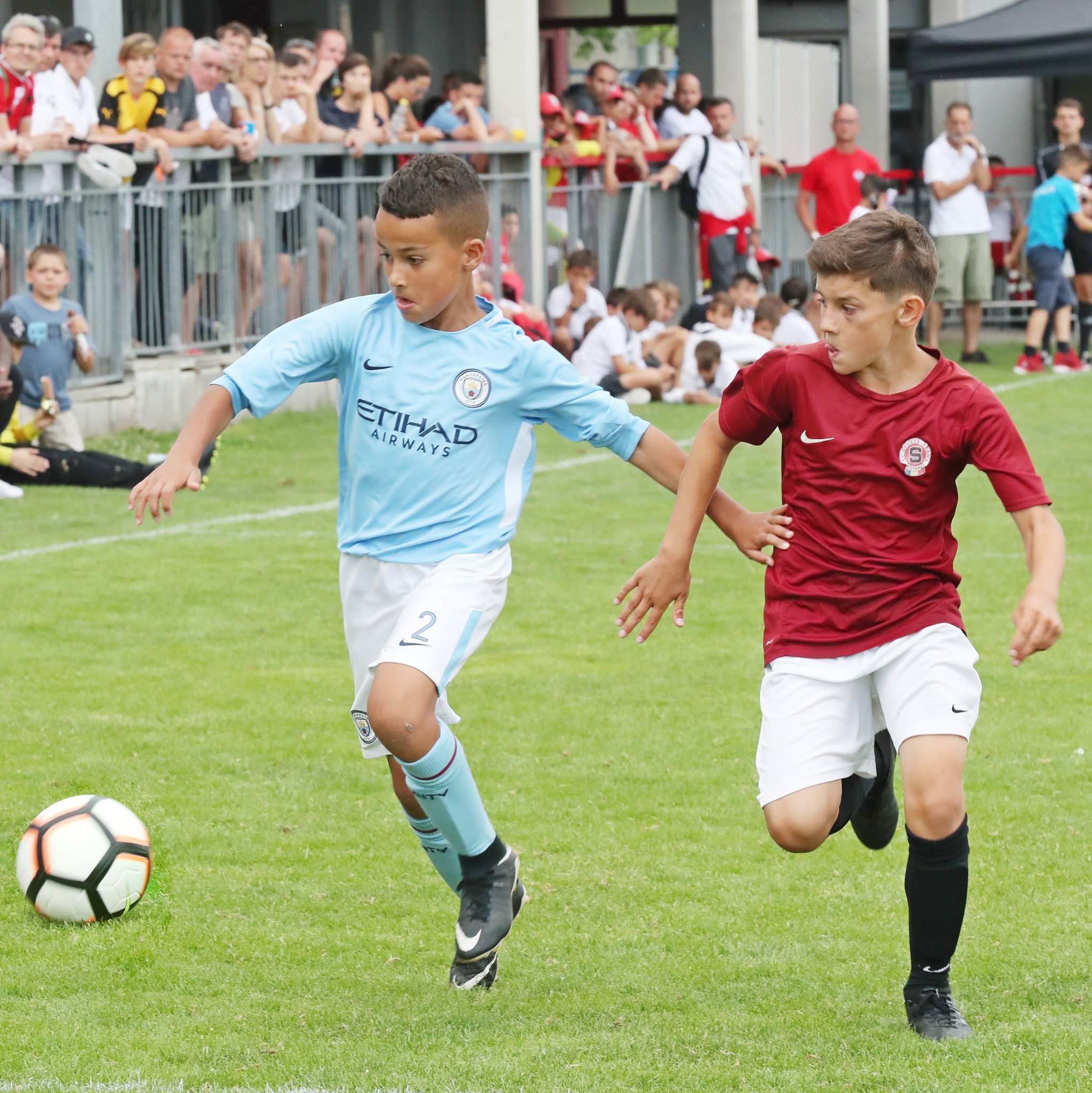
[389, 98, 409, 135]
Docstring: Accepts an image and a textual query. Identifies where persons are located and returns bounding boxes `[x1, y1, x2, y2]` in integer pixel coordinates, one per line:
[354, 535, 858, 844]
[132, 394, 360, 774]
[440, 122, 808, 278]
[127, 151, 794, 994]
[614, 210, 1069, 1040]
[0, 0, 1092, 493]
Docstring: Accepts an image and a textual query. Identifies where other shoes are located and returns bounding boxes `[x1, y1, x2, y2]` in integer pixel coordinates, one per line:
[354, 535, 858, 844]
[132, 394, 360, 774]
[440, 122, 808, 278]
[197, 436, 219, 489]
[616, 388, 651, 405]
[961, 349, 989, 363]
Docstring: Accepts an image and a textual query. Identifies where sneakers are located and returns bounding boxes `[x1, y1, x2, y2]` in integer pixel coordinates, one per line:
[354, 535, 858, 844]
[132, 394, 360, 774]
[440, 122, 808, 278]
[904, 993, 974, 1042]
[1012, 350, 1092, 374]
[455, 843, 520, 962]
[449, 951, 498, 991]
[850, 729, 898, 849]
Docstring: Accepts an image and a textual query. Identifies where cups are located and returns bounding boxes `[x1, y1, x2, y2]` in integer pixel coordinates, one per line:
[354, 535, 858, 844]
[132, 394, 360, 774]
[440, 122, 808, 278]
[886, 188, 898, 206]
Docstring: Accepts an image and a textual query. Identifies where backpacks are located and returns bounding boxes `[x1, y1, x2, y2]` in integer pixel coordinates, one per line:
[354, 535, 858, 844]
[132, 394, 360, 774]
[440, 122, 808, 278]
[678, 136, 744, 220]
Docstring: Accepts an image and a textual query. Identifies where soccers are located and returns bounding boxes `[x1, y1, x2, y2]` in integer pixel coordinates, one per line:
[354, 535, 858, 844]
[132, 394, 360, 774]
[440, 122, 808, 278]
[15, 794, 154, 923]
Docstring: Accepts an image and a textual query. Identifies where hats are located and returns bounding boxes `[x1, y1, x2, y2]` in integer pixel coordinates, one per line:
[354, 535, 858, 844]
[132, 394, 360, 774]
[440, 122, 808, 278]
[541, 92, 563, 114]
[61, 27, 95, 50]
